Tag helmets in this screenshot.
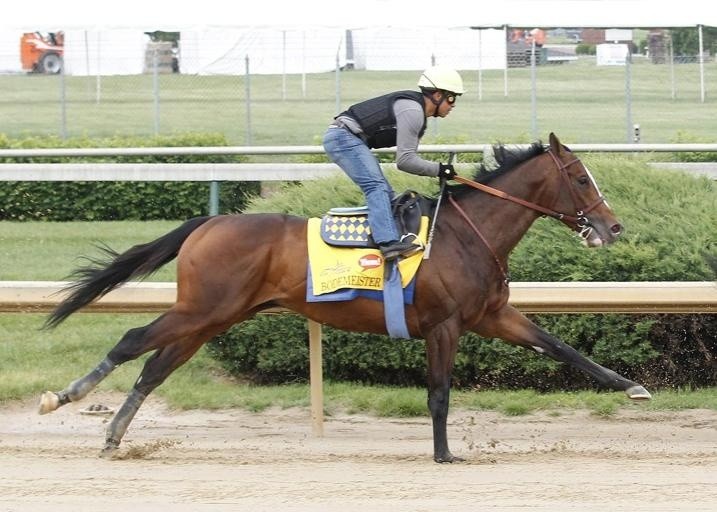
[418,65,467,97]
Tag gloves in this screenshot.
[441,165,458,180]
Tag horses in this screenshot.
[36,131,654,464]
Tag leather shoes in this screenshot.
[379,239,420,261]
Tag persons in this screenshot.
[322,67,465,264]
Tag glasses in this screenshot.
[445,92,456,103]
